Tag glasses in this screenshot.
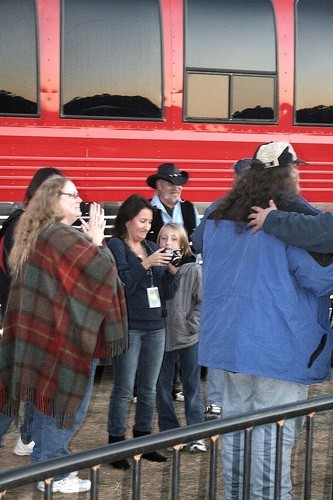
[59,192,80,199]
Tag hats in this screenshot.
[147,163,189,189]
[255,141,308,169]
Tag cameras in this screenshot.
[163,249,181,266]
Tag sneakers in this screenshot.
[13,435,36,455]
[36,471,92,494]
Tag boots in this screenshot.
[108,434,131,470]
[133,429,168,462]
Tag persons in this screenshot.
[196,140,333,500]
[0,156,252,470]
[246,200,333,255]
[0,177,127,492]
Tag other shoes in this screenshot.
[172,443,189,453]
[172,391,185,402]
[188,438,208,453]
